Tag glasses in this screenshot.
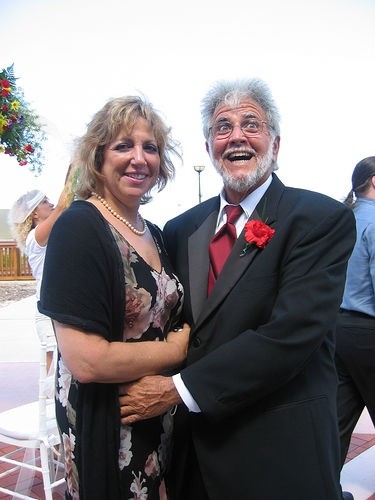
[209,118,274,139]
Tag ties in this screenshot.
[208,205,245,301]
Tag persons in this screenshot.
[118,76,357,500]
[37,95,191,500]
[7,154,79,374]
[334,158,375,500]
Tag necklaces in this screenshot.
[91,191,148,236]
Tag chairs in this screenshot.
[0,314,66,500]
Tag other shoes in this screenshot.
[341,491,354,500]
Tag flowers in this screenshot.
[239,196,276,258]
[0,61,50,178]
